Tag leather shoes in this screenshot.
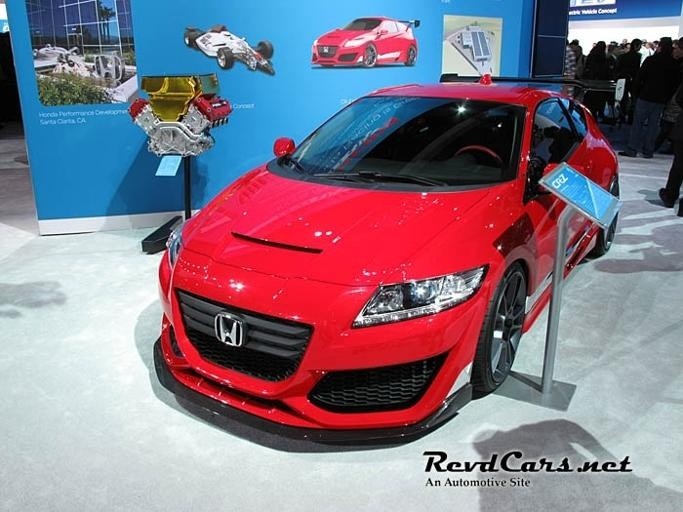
[659,188,674,207]
[618,152,653,158]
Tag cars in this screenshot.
[182,23,278,77]
[151,70,623,449]
[308,14,421,69]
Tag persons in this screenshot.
[564,37,683,157]
[58,54,74,65]
[660,90,683,207]
